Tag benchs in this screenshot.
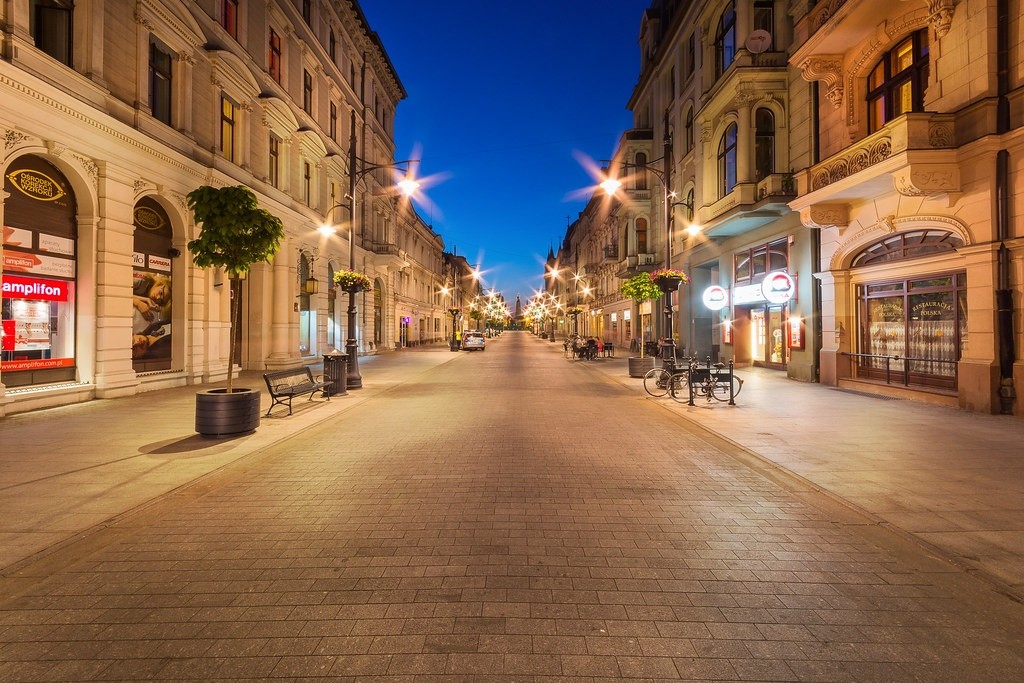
[262,366,334,416]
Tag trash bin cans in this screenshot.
[321,348,350,398]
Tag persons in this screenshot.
[563,334,604,360]
[456,330,462,350]
[132,272,172,360]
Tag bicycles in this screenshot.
[666,357,744,404]
[644,357,711,398]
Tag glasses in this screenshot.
[159,285,165,306]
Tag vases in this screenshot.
[653,276,682,292]
[341,285,361,293]
[449,309,459,314]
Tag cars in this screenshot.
[463,332,486,351]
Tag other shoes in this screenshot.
[587,358,589,360]
[591,358,592,359]
[578,355,583,358]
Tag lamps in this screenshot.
[295,254,318,298]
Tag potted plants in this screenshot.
[185,185,287,435]
[619,271,666,377]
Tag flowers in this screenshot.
[447,306,462,311]
[566,306,583,312]
[333,269,373,293]
[645,269,693,286]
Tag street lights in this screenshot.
[321,159,421,391]
[549,264,590,341]
[441,265,481,353]
[600,159,699,389]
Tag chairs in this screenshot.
[566,340,614,360]
